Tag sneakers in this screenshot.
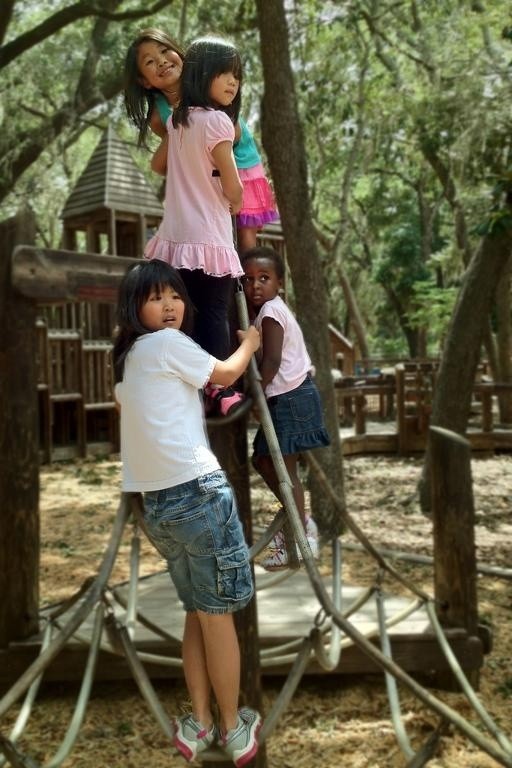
[201,381,246,417]
[263,512,321,571]
[172,707,263,768]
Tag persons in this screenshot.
[108,257,268,766]
[122,26,281,255]
[142,32,248,419]
[239,245,334,570]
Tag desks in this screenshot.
[335,372,512,432]
